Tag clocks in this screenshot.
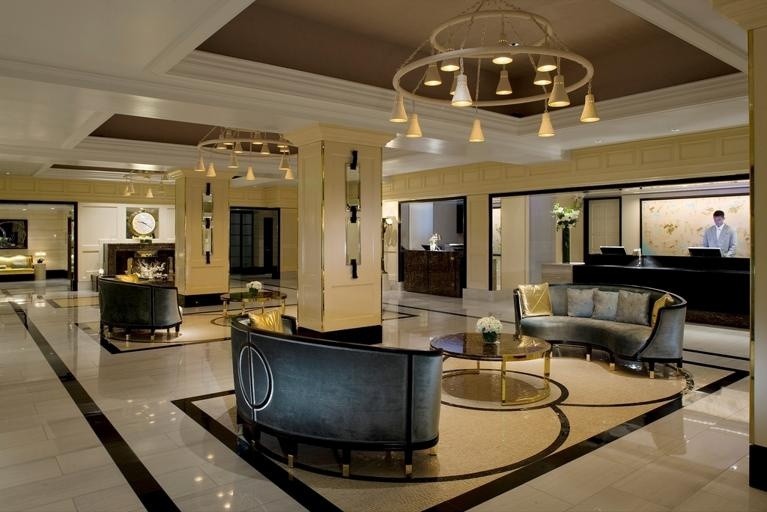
[126,207,156,238]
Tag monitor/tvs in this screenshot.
[600,246,627,255]
[688,247,722,257]
[422,244,430,249]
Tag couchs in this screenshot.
[0,253,33,278]
[98,275,182,341]
[514,281,690,381]
[228,309,443,477]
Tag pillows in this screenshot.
[591,289,618,319]
[115,274,137,285]
[613,288,651,325]
[246,308,283,333]
[517,282,554,319]
[650,292,672,327]
[566,286,599,317]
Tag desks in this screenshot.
[540,261,584,285]
[34,263,46,282]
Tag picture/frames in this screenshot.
[0,218,28,249]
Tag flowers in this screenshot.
[245,280,262,292]
[548,202,580,229]
[35,251,46,263]
[475,316,500,333]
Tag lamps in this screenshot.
[387,0,600,146]
[120,169,164,200]
[195,123,297,184]
[381,217,403,274]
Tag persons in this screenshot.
[703,211,737,257]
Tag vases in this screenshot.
[484,331,496,341]
[248,287,257,295]
[561,228,569,263]
